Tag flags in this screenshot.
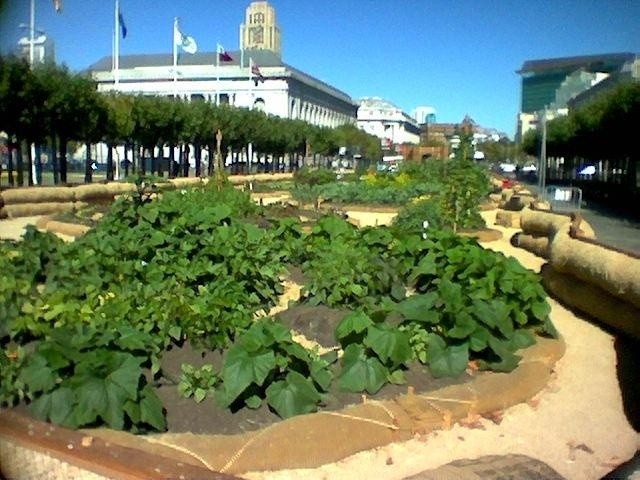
[120,12,127,38]
[175,25,197,55]
[218,45,233,62]
[53,0,61,13]
[252,62,264,86]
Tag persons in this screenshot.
[40,151,49,173]
[200,144,210,178]
[183,159,190,177]
[256,157,299,173]
[172,161,182,177]
